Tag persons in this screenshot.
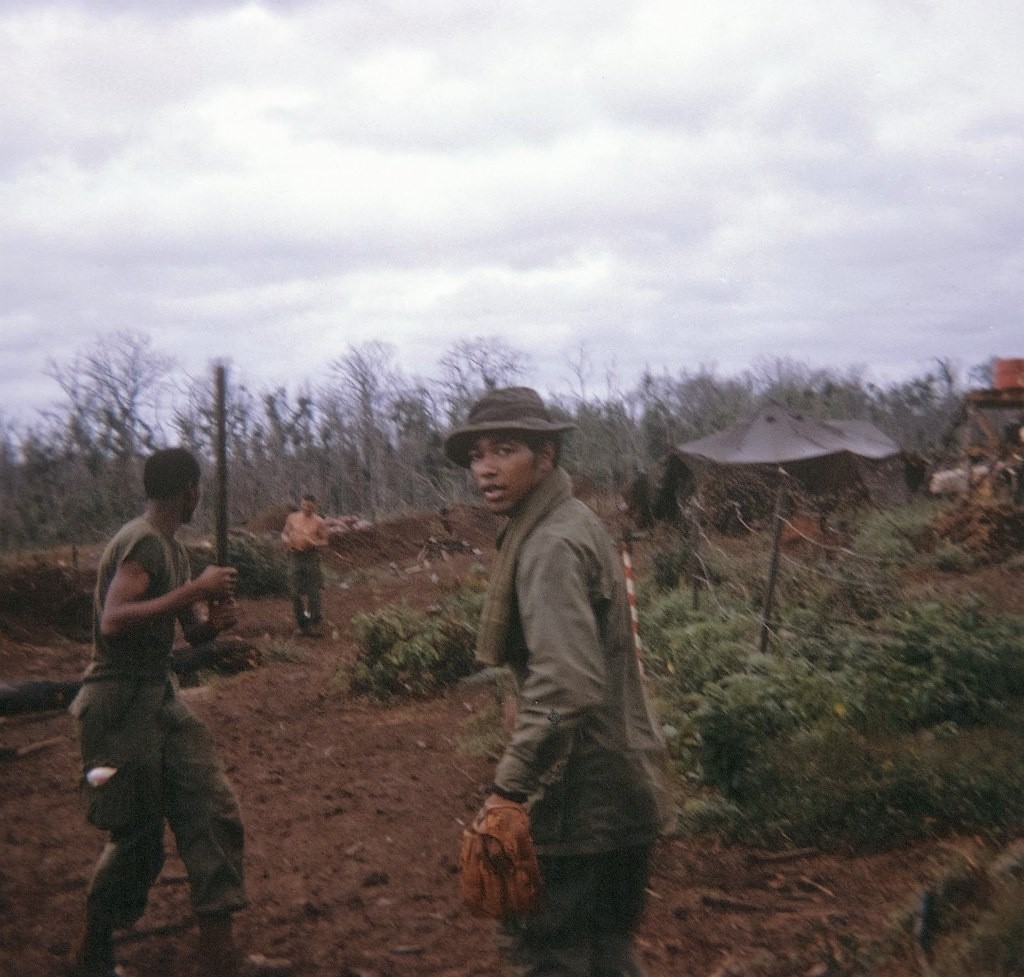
[280,495,330,639]
[445,389,674,976]
[73,448,291,976]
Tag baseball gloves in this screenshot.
[457,801,542,919]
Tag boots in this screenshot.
[73,892,141,977]
[196,913,292,977]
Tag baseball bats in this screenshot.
[214,362,228,568]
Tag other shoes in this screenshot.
[300,617,324,637]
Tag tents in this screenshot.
[676,395,902,467]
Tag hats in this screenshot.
[444,387,579,469]
